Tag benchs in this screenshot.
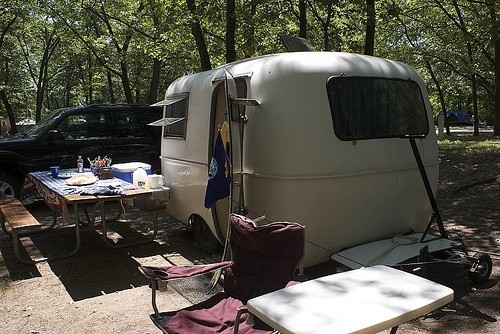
[119,198,168,245]
[0,198,41,264]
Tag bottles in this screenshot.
[98,167,113,179]
[77,156,83,173]
[132,167,148,190]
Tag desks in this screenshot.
[20,168,169,262]
[247,264,454,334]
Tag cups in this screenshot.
[50,166,60,178]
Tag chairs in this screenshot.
[138,213,305,334]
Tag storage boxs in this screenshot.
[110,162,152,184]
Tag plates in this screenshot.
[65,178,98,185]
[57,173,76,178]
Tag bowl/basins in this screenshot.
[149,174,165,188]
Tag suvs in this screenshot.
[0,103,163,201]
